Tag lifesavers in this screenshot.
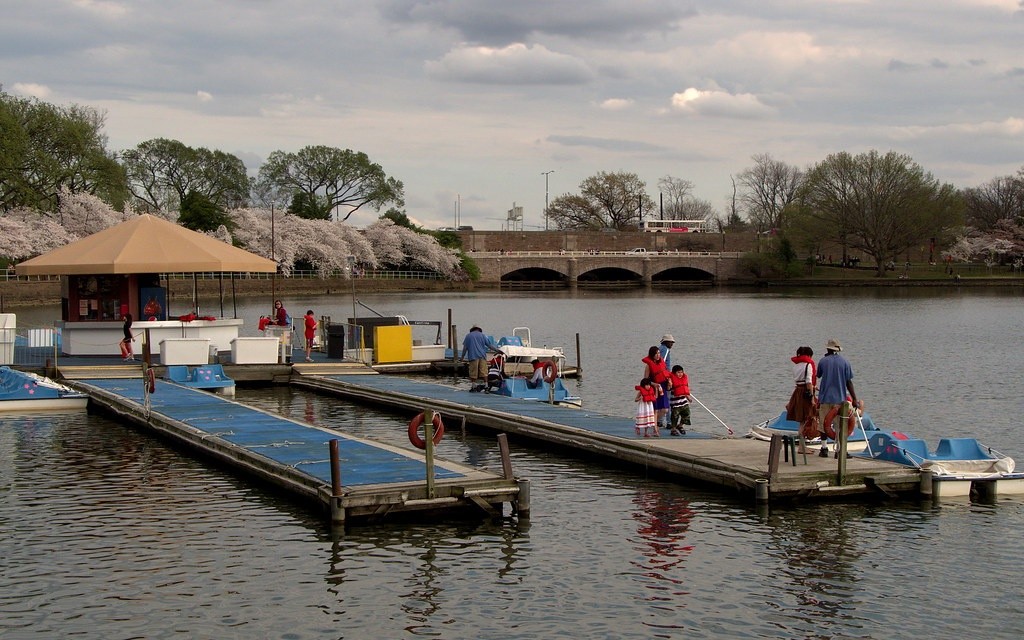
[542,360,557,383]
[148,368,155,393]
[823,405,855,441]
[408,411,444,449]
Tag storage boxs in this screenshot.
[229,337,281,364]
[158,338,211,365]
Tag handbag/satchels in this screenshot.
[802,405,821,440]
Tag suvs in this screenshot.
[456,225,473,232]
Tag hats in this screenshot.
[825,338,843,352]
[470,324,482,332]
[531,359,539,364]
[660,334,675,343]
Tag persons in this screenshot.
[304,310,320,363]
[815,253,861,269]
[528,360,550,385]
[634,335,693,438]
[658,247,742,255]
[121,313,136,361]
[559,248,601,255]
[885,255,1014,280]
[460,324,503,393]
[785,338,870,459]
[7,262,14,280]
[272,300,287,326]
[470,247,512,255]
[488,357,510,391]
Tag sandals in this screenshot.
[671,425,685,436]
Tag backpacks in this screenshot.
[280,308,291,327]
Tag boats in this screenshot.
[847,431,1024,496]
[488,345,581,408]
[748,394,881,453]
[0,365,89,410]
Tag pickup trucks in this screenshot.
[625,247,658,256]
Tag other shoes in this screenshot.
[469,388,477,392]
[834,451,852,459]
[798,446,815,454]
[818,447,828,457]
[653,430,660,437]
[304,357,314,363]
[657,421,672,429]
[123,353,134,361]
[485,388,489,394]
[643,433,650,438]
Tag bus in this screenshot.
[637,219,706,233]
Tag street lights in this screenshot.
[541,170,555,231]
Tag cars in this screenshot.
[763,229,787,235]
[438,227,456,232]
